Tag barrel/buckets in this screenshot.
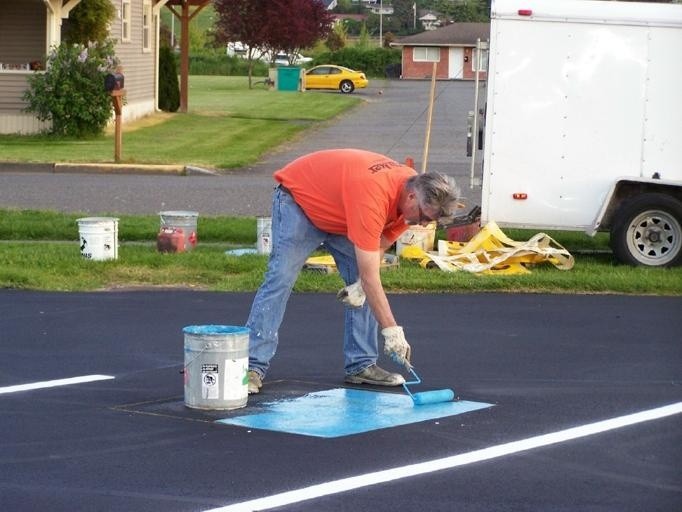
[179,324,251,413]
[74,215,118,264]
[447,220,483,243]
[253,214,274,255]
[157,210,198,253]
[394,220,438,259]
[276,65,301,92]
[268,67,277,91]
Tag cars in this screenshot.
[272,53,313,66]
[304,64,368,94]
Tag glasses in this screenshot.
[417,204,433,223]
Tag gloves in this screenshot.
[381,326,411,365]
[337,278,366,308]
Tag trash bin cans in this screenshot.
[277,67,300,92]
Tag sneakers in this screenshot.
[345,363,405,386]
[248,370,263,394]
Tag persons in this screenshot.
[243,147,460,394]
